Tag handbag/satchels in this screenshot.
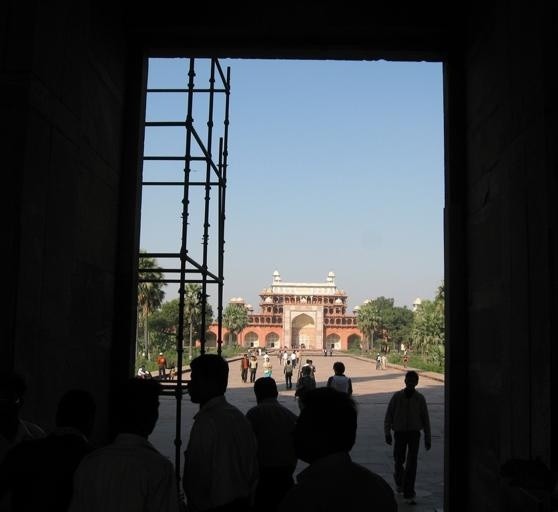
[295,383,305,396]
[264,370,269,374]
[287,372,292,376]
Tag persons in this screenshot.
[184,353,255,512]
[1,364,47,455]
[328,361,351,397]
[384,370,431,502]
[330,347,332,356]
[0,386,99,512]
[240,346,316,408]
[246,376,301,512]
[381,355,387,370]
[324,347,327,356]
[138,365,152,379]
[166,361,175,380]
[277,388,400,511]
[68,376,184,510]
[375,352,384,370]
[156,352,168,380]
